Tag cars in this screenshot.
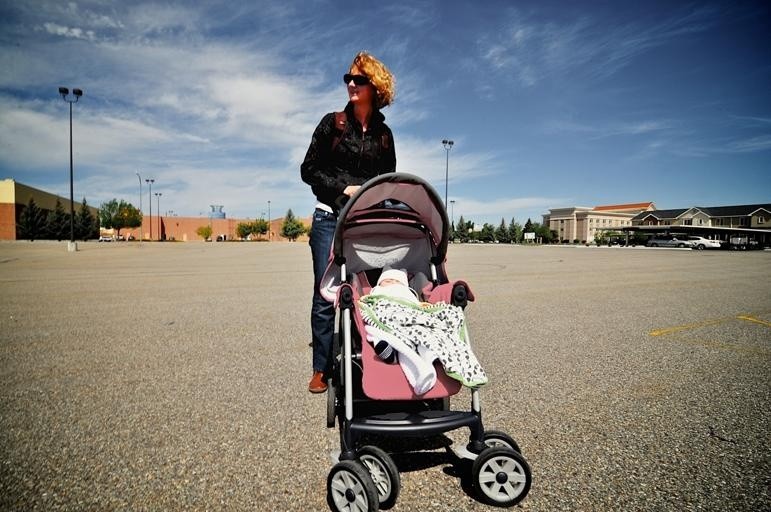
[100,234,135,242]
[448,238,484,243]
[646,233,758,250]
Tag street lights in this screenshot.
[442,140,454,211]
[169,210,179,240]
[268,200,271,241]
[59,86,84,253]
[247,212,266,240]
[146,179,155,242]
[450,200,456,242]
[136,173,142,244]
[156,193,162,241]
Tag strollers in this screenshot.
[319,172,532,511]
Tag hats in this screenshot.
[377,266,409,287]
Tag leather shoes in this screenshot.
[309,371,328,393]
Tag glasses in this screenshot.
[343,73,370,86]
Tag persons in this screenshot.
[364,263,419,365]
[301,51,397,394]
